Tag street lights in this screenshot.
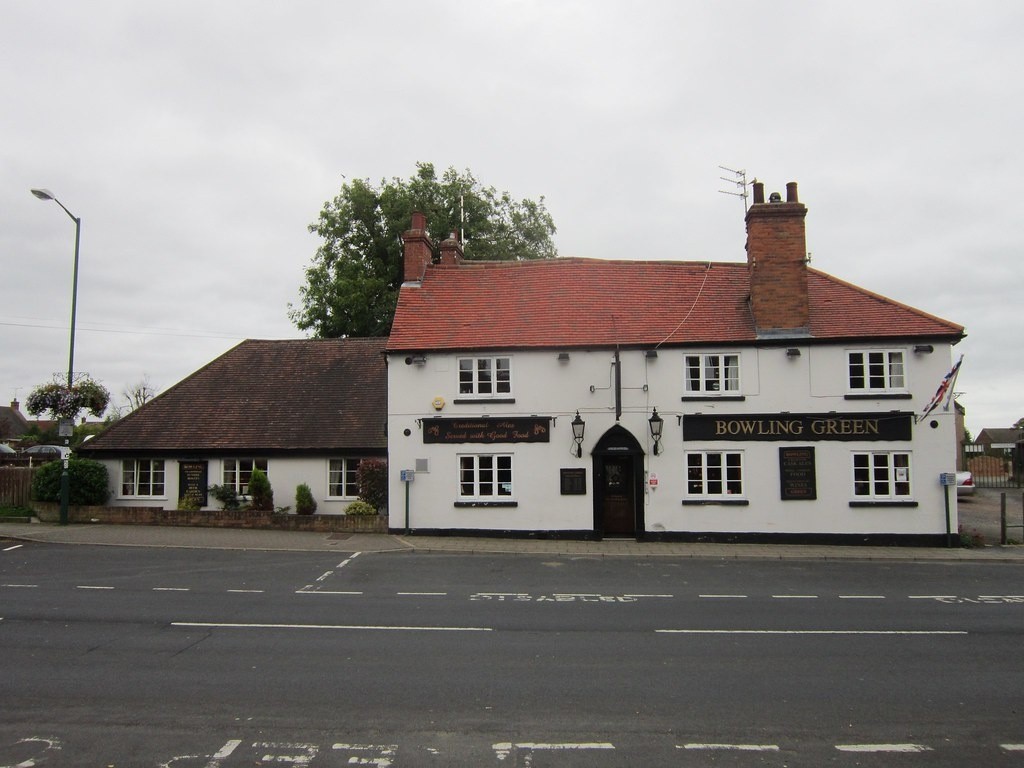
[31,186,81,524]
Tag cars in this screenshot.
[956,471,975,496]
[19,445,64,459]
[0,444,17,459]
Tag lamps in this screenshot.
[571,409,586,458]
[648,406,664,456]
[645,350,658,359]
[785,349,802,359]
[410,355,427,367]
[558,353,570,362]
[913,345,934,354]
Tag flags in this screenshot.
[920,363,960,421]
